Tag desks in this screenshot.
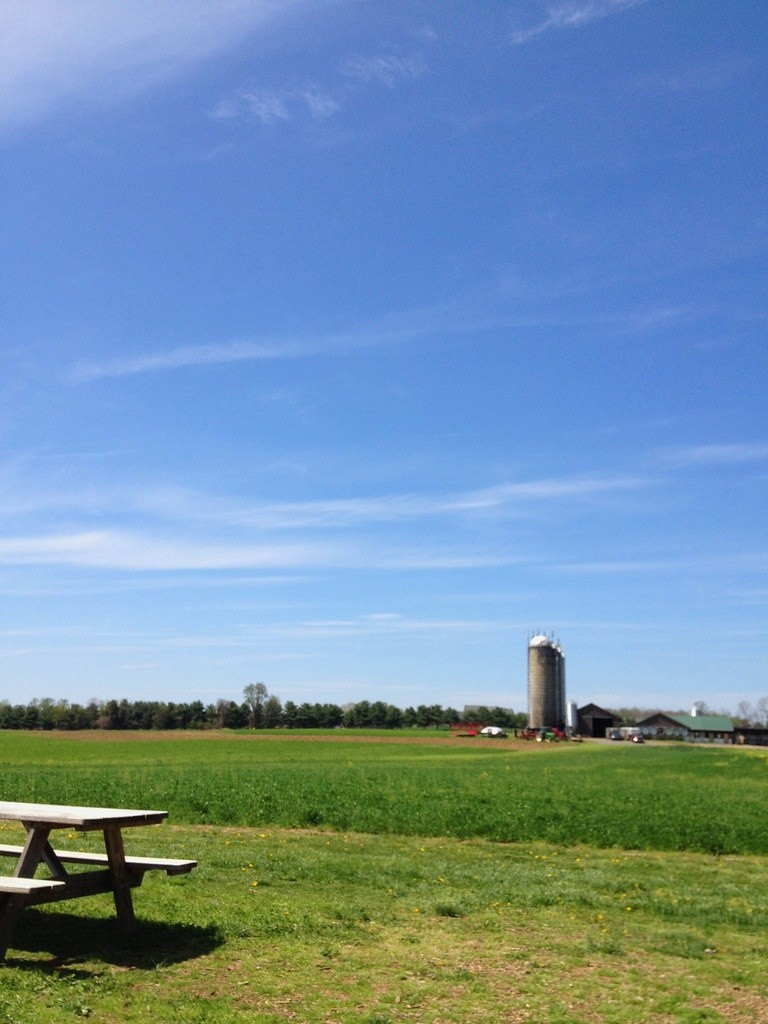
[0,800,198,964]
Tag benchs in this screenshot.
[0,844,198,876]
[0,876,66,894]
